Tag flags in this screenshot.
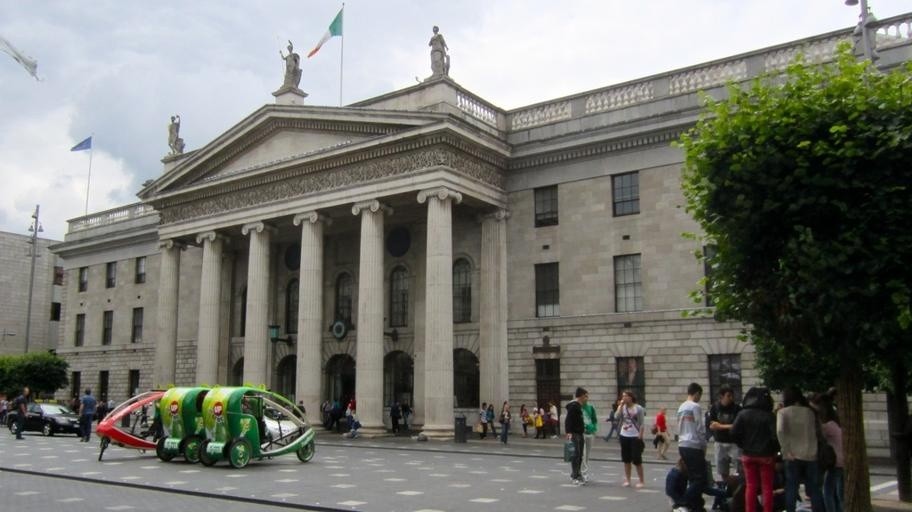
[308,6,344,60]
[70,137,92,152]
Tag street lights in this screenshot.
[22,198,47,354]
[0,332,19,337]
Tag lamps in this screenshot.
[268,322,293,346]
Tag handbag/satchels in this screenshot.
[499,410,504,424]
[476,423,484,433]
[819,445,836,464]
[652,427,658,434]
[564,441,577,464]
[524,416,532,424]
[398,418,405,425]
[536,416,543,428]
[346,409,351,416]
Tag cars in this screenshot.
[7,400,81,438]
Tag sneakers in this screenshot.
[601,436,609,442]
[570,476,646,488]
[15,436,24,439]
[522,432,559,440]
[657,454,667,460]
[80,436,90,442]
[480,435,501,440]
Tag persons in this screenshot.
[564,386,587,486]
[655,405,672,461]
[169,113,181,154]
[478,399,559,446]
[388,400,403,434]
[293,399,306,422]
[318,396,361,438]
[428,26,450,76]
[664,383,849,511]
[401,400,412,431]
[601,403,621,443]
[278,39,301,88]
[580,390,599,483]
[615,388,646,489]
[0,386,115,443]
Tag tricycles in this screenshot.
[90,383,320,471]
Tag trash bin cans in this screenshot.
[454,416,466,443]
